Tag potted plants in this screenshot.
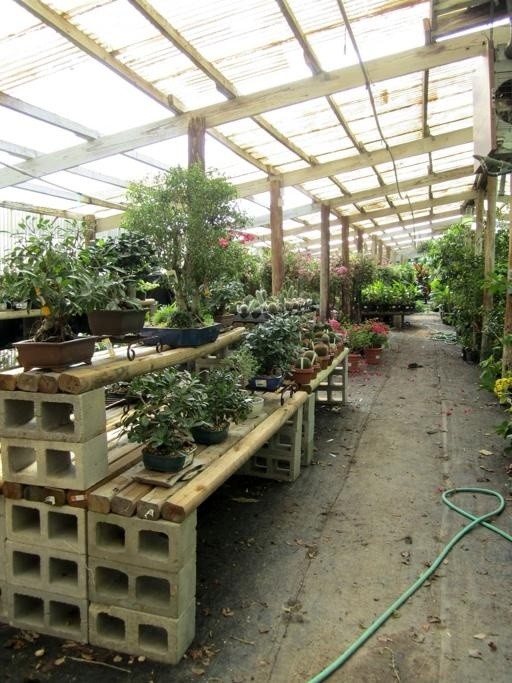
[429,226,490,366]
[0,153,416,473]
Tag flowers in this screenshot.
[494,377,512,440]
